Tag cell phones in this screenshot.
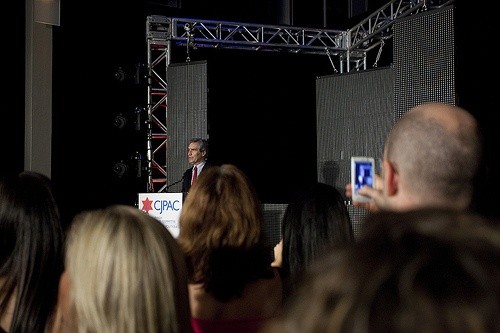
[350,156,376,203]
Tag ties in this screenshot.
[192,167,197,184]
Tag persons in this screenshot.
[289,102,500,333]
[0,164,500,333]
[181,138,211,202]
